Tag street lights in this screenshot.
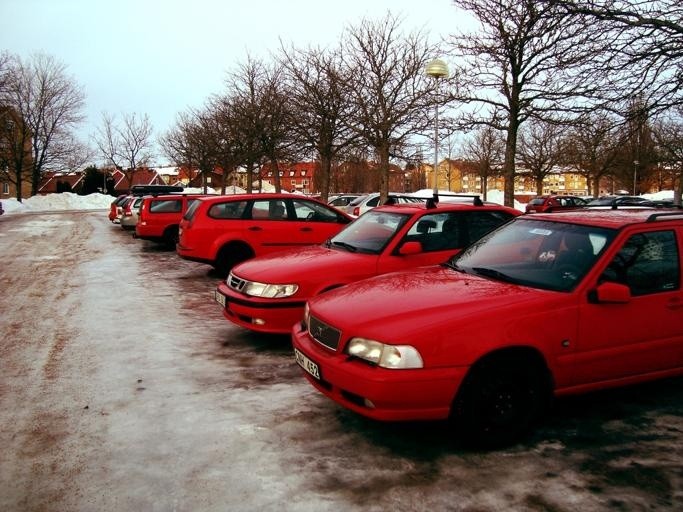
[423,60,448,200]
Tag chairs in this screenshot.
[545,231,589,271]
[269,205,286,219]
